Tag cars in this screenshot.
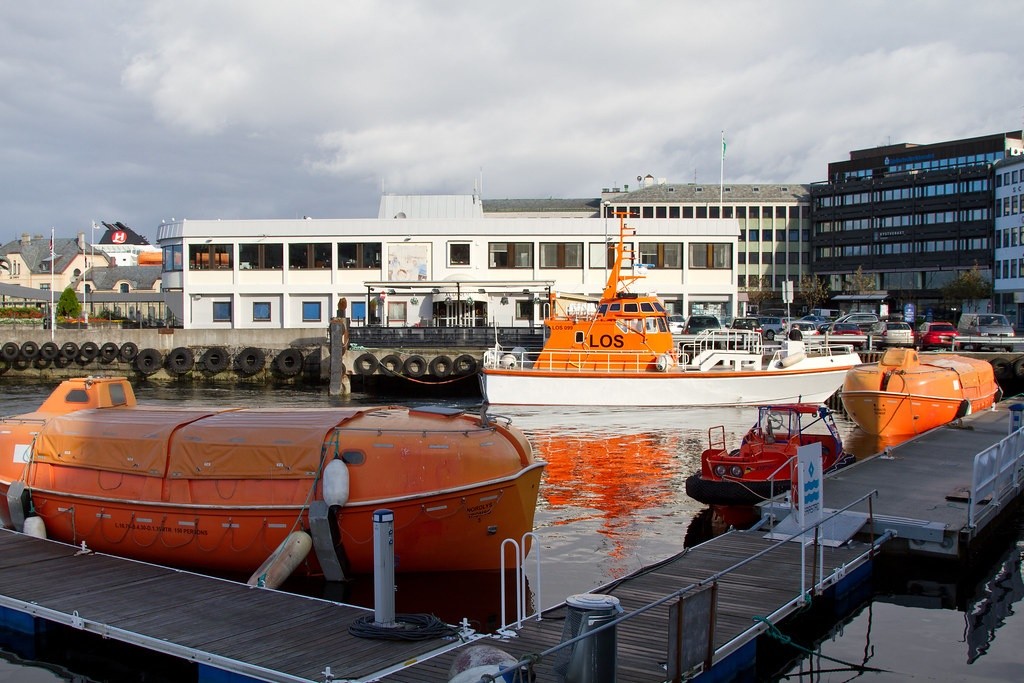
[824,323,864,351]
[726,317,765,348]
[779,322,822,352]
[914,322,960,352]
[742,313,830,341]
[867,320,914,350]
[667,314,685,334]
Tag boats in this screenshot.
[0,316,545,584]
[481,211,862,407]
[687,397,856,529]
[839,346,1002,438]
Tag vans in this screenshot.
[957,312,1016,352]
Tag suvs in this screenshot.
[818,312,882,336]
[677,314,722,350]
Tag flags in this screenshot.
[50,236,56,256]
[723,137,727,161]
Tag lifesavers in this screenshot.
[356,354,379,376]
[240,347,266,374]
[431,355,454,378]
[41,342,59,359]
[2,342,19,360]
[136,349,163,374]
[380,355,403,377]
[454,355,477,376]
[168,347,194,374]
[80,341,99,359]
[121,342,138,360]
[22,341,39,358]
[404,355,427,378]
[61,342,79,359]
[203,347,229,373]
[101,342,119,360]
[276,348,304,376]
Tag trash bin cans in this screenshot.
[552,593,625,683]
